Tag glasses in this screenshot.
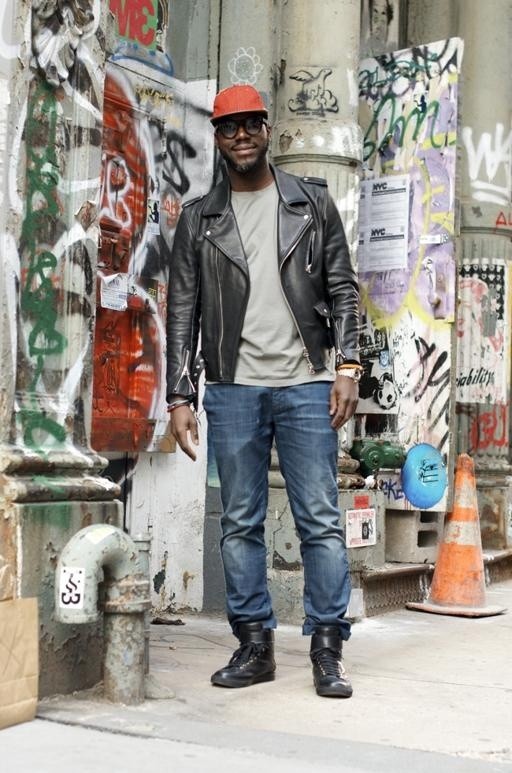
[213,112,271,140]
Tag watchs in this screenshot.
[336,369,362,383]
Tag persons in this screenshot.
[165,83,362,699]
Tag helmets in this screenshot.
[210,84,269,127]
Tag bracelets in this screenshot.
[337,363,365,377]
[167,399,189,413]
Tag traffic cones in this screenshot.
[404,452,507,617]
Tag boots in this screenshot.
[309,627,353,698]
[211,622,276,688]
